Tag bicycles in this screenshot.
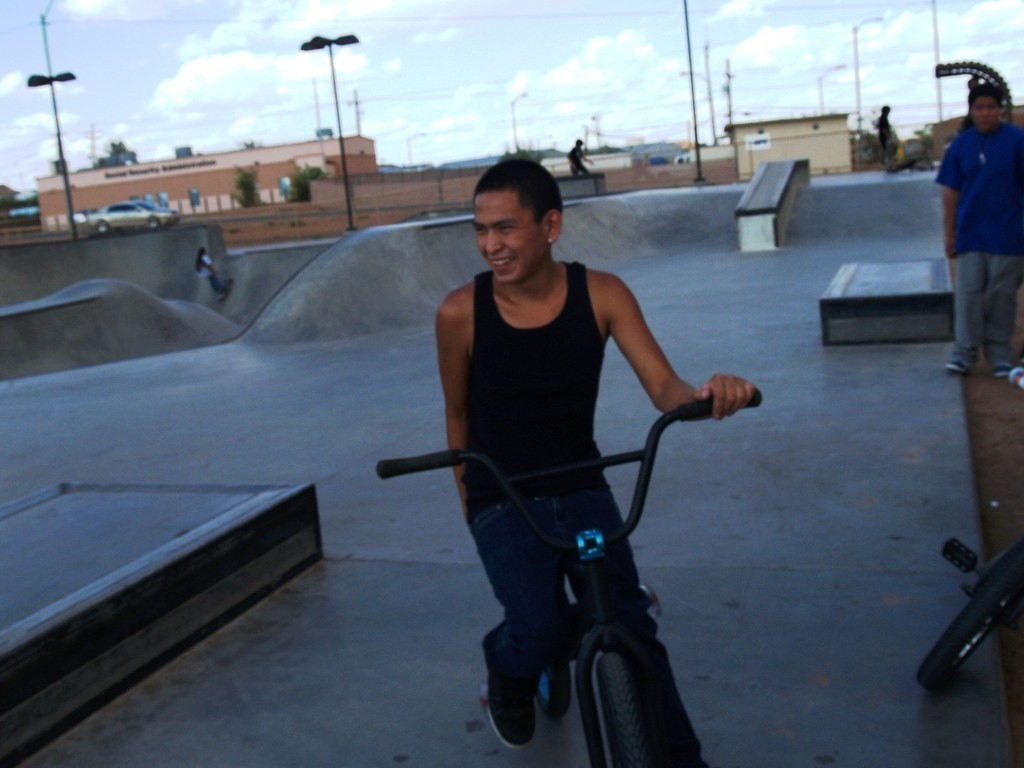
[375,389,764,764]
[911,530,1024,693]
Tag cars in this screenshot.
[86,198,181,234]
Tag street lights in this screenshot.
[302,35,361,230]
[28,72,78,241]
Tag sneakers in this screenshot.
[486,660,538,750]
[993,362,1012,378]
[945,360,967,374]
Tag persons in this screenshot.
[876,106,892,167]
[567,140,593,175]
[935,84,1024,376]
[195,247,231,301]
[435,159,753,768]
